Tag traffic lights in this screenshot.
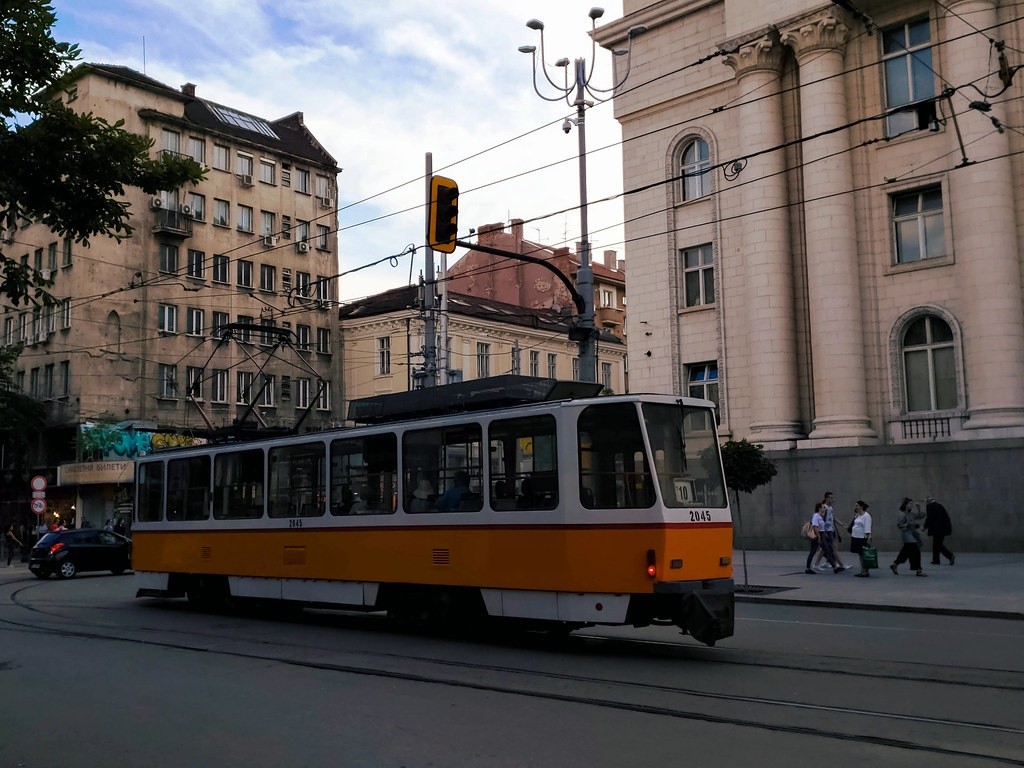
[429,175,460,253]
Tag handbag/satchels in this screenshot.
[800,521,817,539]
[848,515,858,534]
[913,532,923,547]
[862,539,879,569]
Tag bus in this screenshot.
[128,323,737,649]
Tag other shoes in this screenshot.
[805,568,817,574]
[890,564,899,575]
[916,569,927,577]
[8,564,14,568]
[854,574,870,577]
[834,566,844,573]
[950,556,955,565]
[931,561,940,564]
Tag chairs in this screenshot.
[287,477,540,517]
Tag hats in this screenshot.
[925,497,935,505]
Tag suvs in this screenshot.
[27,528,133,580]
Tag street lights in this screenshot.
[517,7,650,384]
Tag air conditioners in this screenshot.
[296,242,309,252]
[35,332,46,343]
[264,236,277,246]
[322,198,333,207]
[179,204,192,214]
[320,299,332,310]
[39,269,50,281]
[24,335,34,346]
[240,175,256,187]
[150,197,166,208]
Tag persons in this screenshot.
[805,491,955,577]
[5,517,126,568]
[229,470,547,519]
